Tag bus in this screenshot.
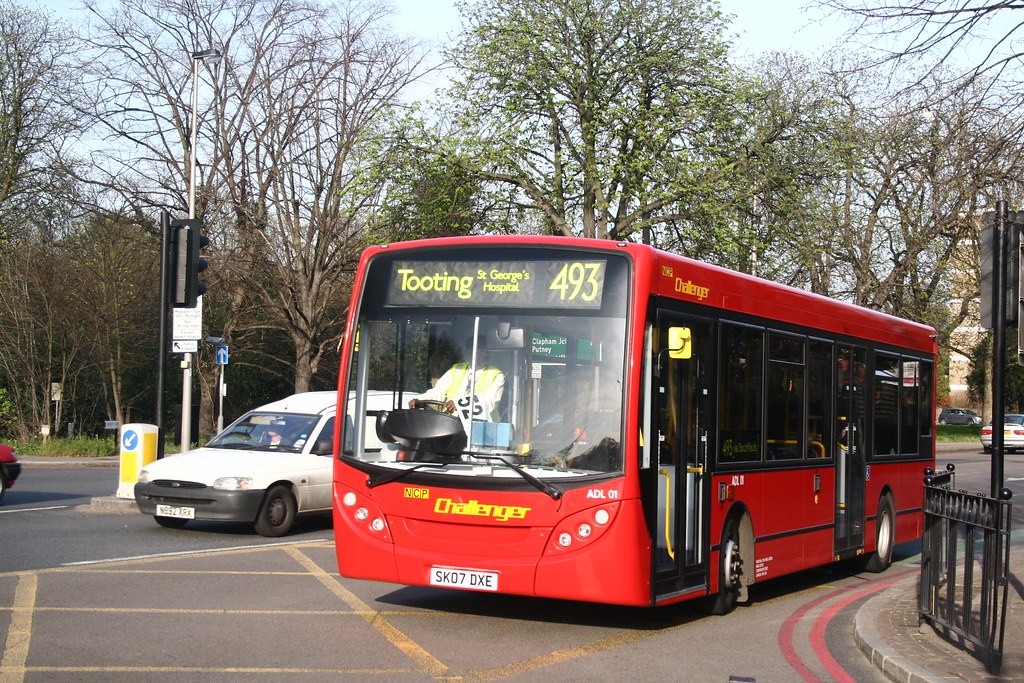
[330,233,939,617]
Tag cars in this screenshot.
[938,408,983,426]
[979,414,1024,455]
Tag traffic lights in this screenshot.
[172,219,209,309]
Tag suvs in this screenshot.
[133,389,421,536]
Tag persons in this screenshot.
[837,350,882,433]
[409,334,505,417]
[260,418,293,451]
